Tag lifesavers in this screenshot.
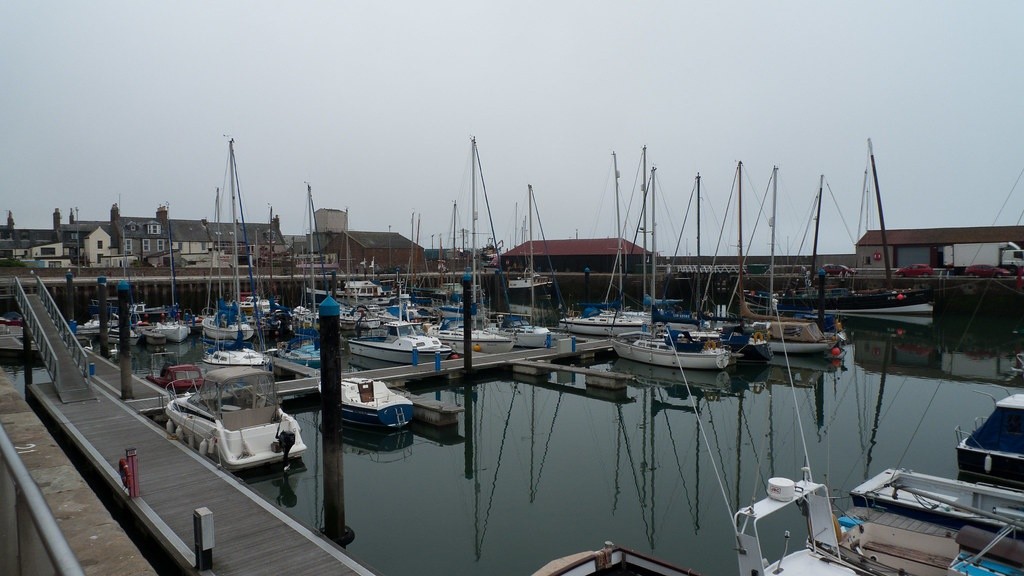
[754,332,763,341]
[302,320,313,330]
[119,459,130,488]
[706,341,716,350]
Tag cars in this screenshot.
[895,264,934,277]
[822,264,858,276]
[965,264,1011,279]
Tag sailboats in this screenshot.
[424,135,517,353]
[609,355,841,551]
[152,220,191,343]
[558,151,653,337]
[202,137,396,372]
[485,184,560,348]
[744,138,934,314]
[611,161,840,369]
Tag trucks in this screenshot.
[942,242,1024,275]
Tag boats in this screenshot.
[0,297,213,394]
[954,386,1024,490]
[348,321,452,364]
[531,541,703,576]
[313,410,414,463]
[165,365,414,472]
[850,468,1024,541]
[733,467,873,576]
